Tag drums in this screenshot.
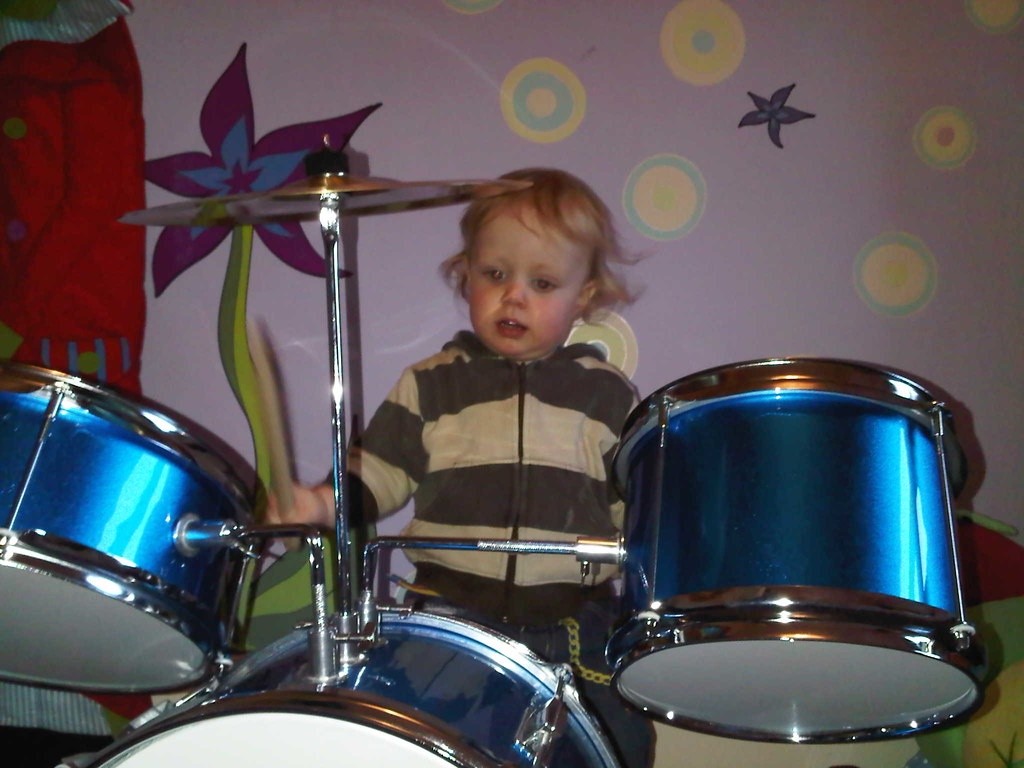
[604,355,991,746]
[64,601,618,768]
[1,359,268,694]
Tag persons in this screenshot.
[267,167,657,768]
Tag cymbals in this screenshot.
[116,171,537,228]
